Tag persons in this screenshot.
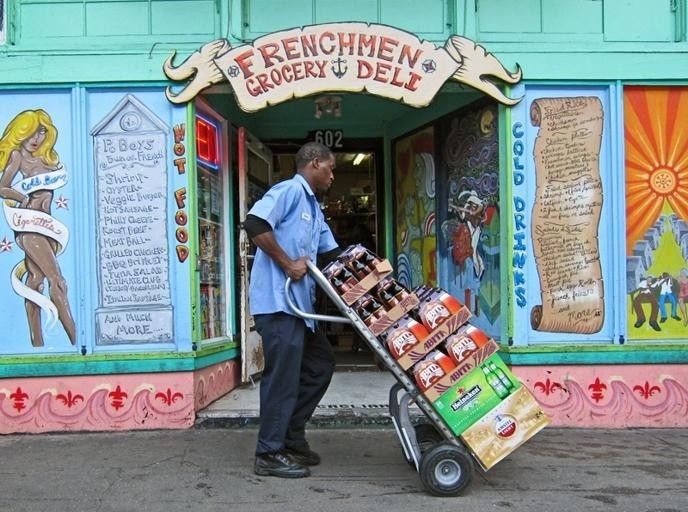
[244,142,343,478]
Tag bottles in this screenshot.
[323,242,410,327]
[479,360,514,400]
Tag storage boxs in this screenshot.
[459,382,550,470]
[432,354,522,436]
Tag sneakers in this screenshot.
[253,446,321,478]
